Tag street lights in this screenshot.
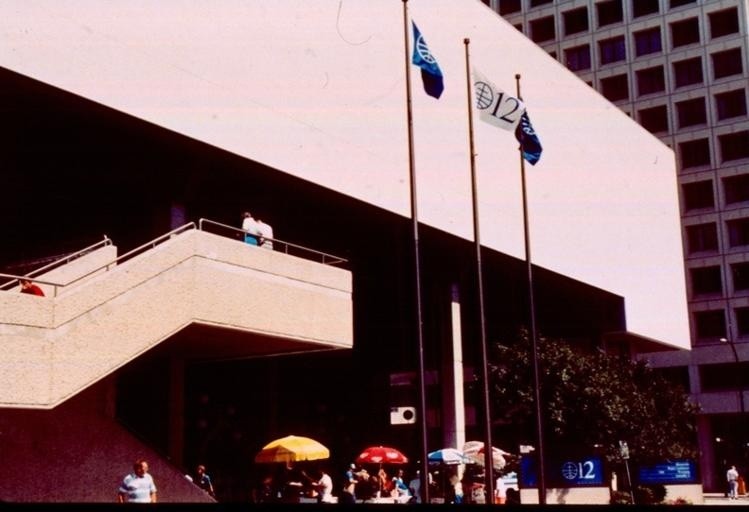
[720,337,745,457]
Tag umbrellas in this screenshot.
[354,444,409,498]
[253,434,332,470]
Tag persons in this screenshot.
[342,463,358,499]
[254,216,275,251]
[354,468,380,499]
[405,464,520,509]
[117,459,159,504]
[310,467,333,498]
[17,276,46,297]
[195,463,216,499]
[375,469,394,496]
[725,464,741,500]
[391,468,413,498]
[279,461,305,497]
[235,210,264,246]
[249,471,275,504]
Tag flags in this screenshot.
[515,103,544,170]
[467,61,522,132]
[410,16,445,101]
